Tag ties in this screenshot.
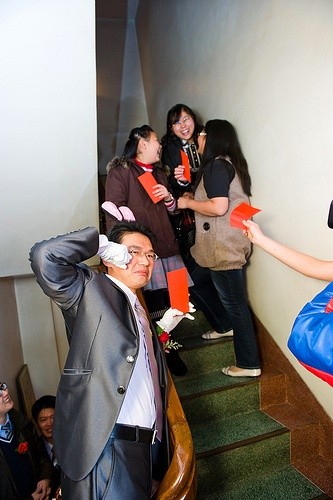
[1,421,12,439]
[135,296,163,443]
[51,446,60,470]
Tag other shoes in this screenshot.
[201,329,234,340]
[221,365,262,378]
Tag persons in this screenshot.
[104,103,263,377]
[0,381,62,500]
[28,201,196,500]
[243,200,333,388]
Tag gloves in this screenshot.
[99,234,132,270]
[156,293,197,334]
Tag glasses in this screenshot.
[0,382,8,397]
[172,116,193,127]
[129,248,160,262]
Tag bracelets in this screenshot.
[164,194,173,202]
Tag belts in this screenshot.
[110,423,158,446]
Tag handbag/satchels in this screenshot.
[174,208,195,249]
